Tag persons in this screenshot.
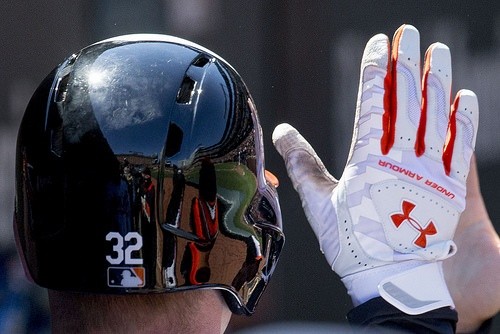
[113,150,219,286]
[443,153,499,334]
[13,25,481,334]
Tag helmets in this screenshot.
[9,31,284,319]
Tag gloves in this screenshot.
[265,22,481,318]
